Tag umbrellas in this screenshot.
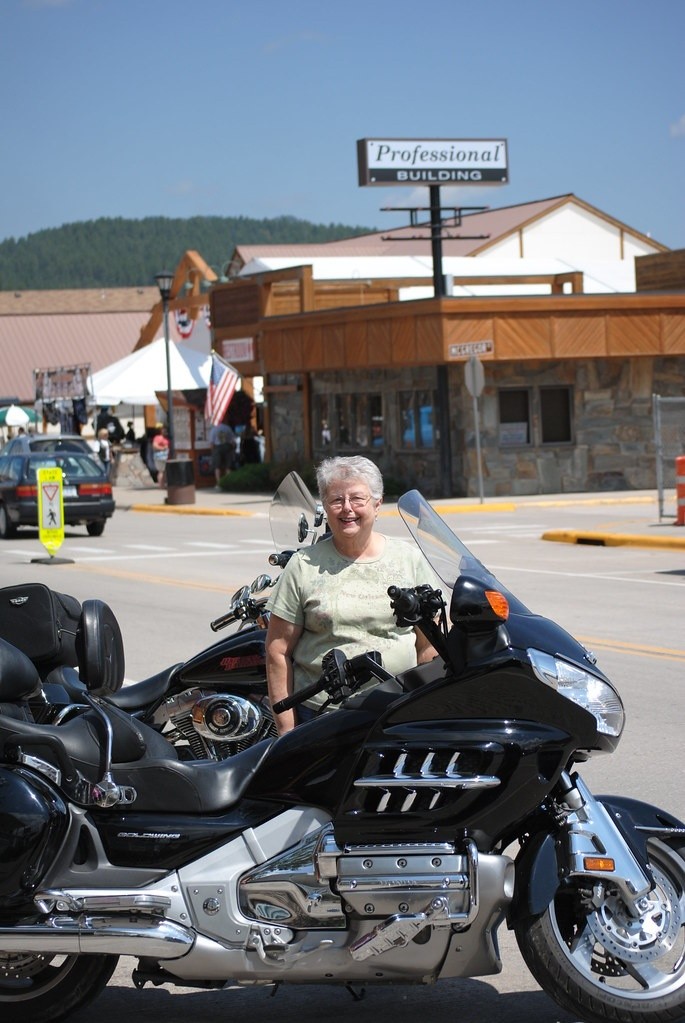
[0,404,44,427]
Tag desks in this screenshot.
[111,447,142,487]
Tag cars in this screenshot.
[345,404,439,450]
[0,433,109,479]
[0,454,116,537]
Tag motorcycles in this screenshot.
[0,470,685,1023]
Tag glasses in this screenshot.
[325,495,373,515]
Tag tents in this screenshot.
[84,337,264,406]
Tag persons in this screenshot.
[126,422,135,446]
[211,418,236,488]
[240,420,265,464]
[265,454,444,737]
[92,405,125,462]
[96,427,114,480]
[152,420,170,488]
[17,427,27,438]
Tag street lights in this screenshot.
[155,262,176,461]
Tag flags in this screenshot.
[204,356,241,428]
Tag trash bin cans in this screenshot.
[163,458,197,504]
